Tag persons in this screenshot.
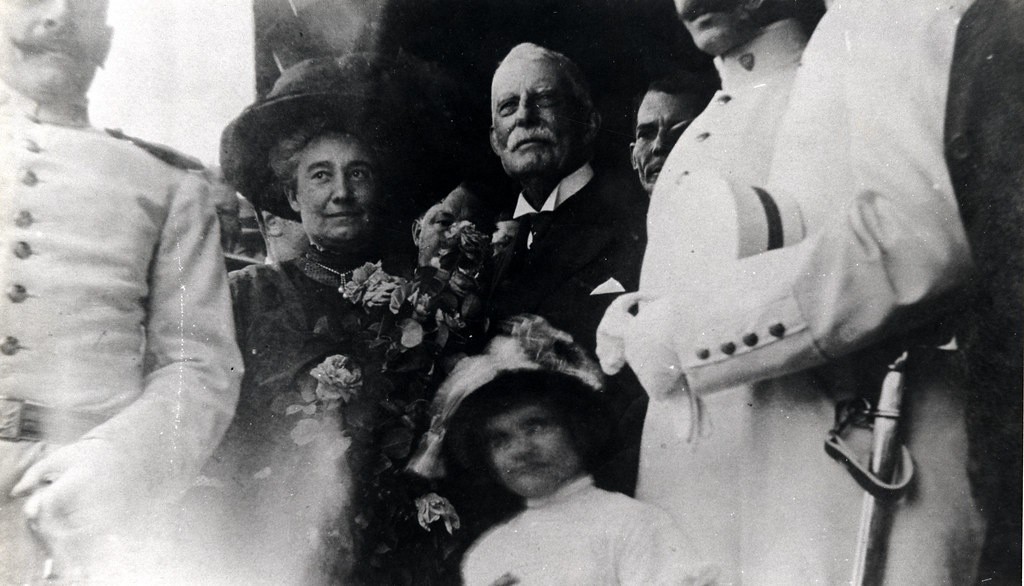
[200,180,254,273]
[0,1,246,586]
[410,316,721,586]
[628,1,811,586]
[218,55,446,586]
[254,205,311,271]
[597,1,991,586]
[399,39,645,586]
[603,64,719,505]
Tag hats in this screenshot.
[405,312,603,478]
[630,172,807,315]
[218,53,420,222]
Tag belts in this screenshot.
[0,391,116,444]
